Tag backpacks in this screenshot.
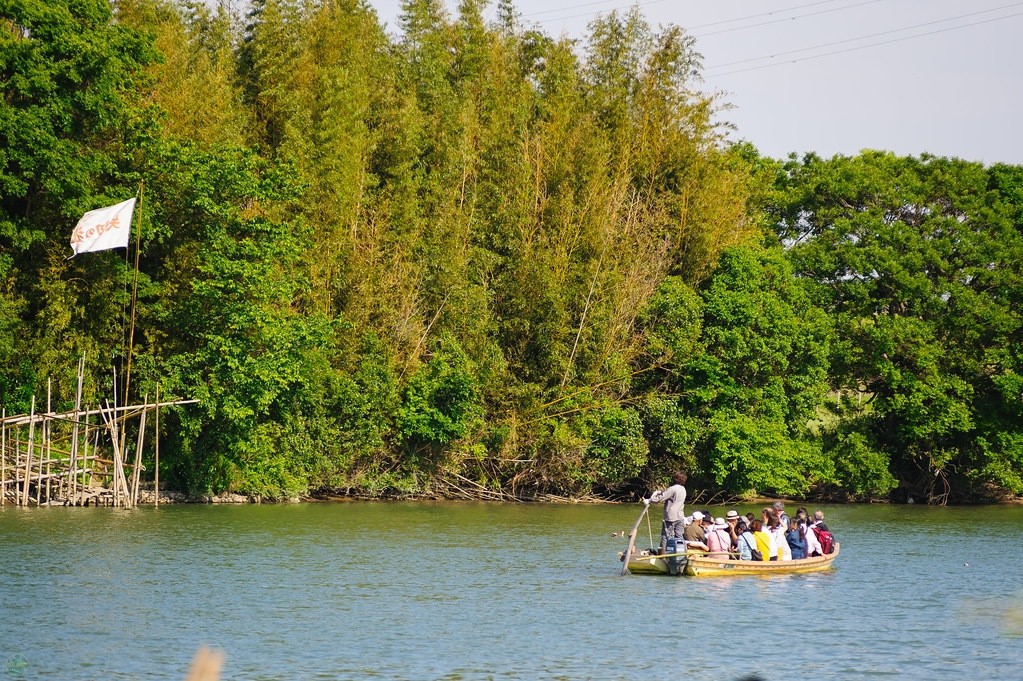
[812,528,834,554]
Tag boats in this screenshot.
[623,541,840,576]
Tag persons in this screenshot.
[683,502,835,562]
[652,472,686,560]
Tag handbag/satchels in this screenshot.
[751,549,763,560]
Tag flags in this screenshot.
[67,198,134,260]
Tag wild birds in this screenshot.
[611,531,625,537]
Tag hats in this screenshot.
[692,511,706,520]
[725,510,739,520]
[703,514,714,523]
[714,518,729,528]
